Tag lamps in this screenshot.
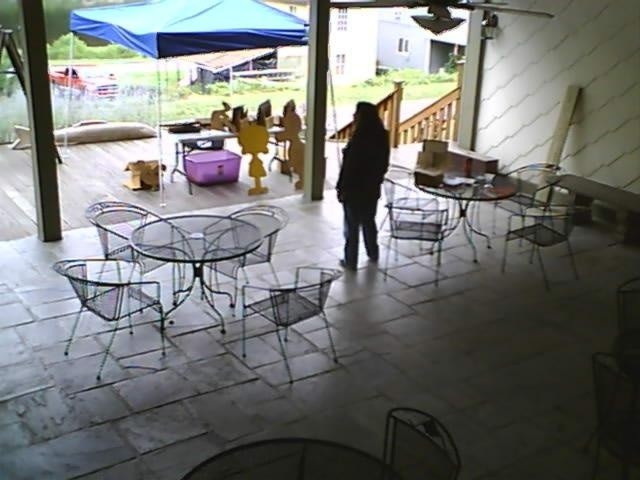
[479,12,504,42]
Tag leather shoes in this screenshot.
[339,258,357,272]
[371,252,380,262]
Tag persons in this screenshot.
[336,101,390,272]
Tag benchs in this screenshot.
[551,173,639,246]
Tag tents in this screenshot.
[64,0,341,207]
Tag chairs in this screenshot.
[382,196,450,289]
[241,266,346,385]
[51,257,167,381]
[614,276,640,336]
[83,199,193,316]
[376,164,422,246]
[200,204,291,318]
[382,407,462,480]
[501,203,581,293]
[491,168,562,266]
[590,351,640,479]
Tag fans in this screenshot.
[328,0,556,37]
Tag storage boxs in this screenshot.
[183,148,242,188]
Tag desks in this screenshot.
[169,124,293,195]
[180,437,406,480]
[129,214,265,336]
[415,170,521,265]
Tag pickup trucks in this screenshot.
[48,63,119,101]
